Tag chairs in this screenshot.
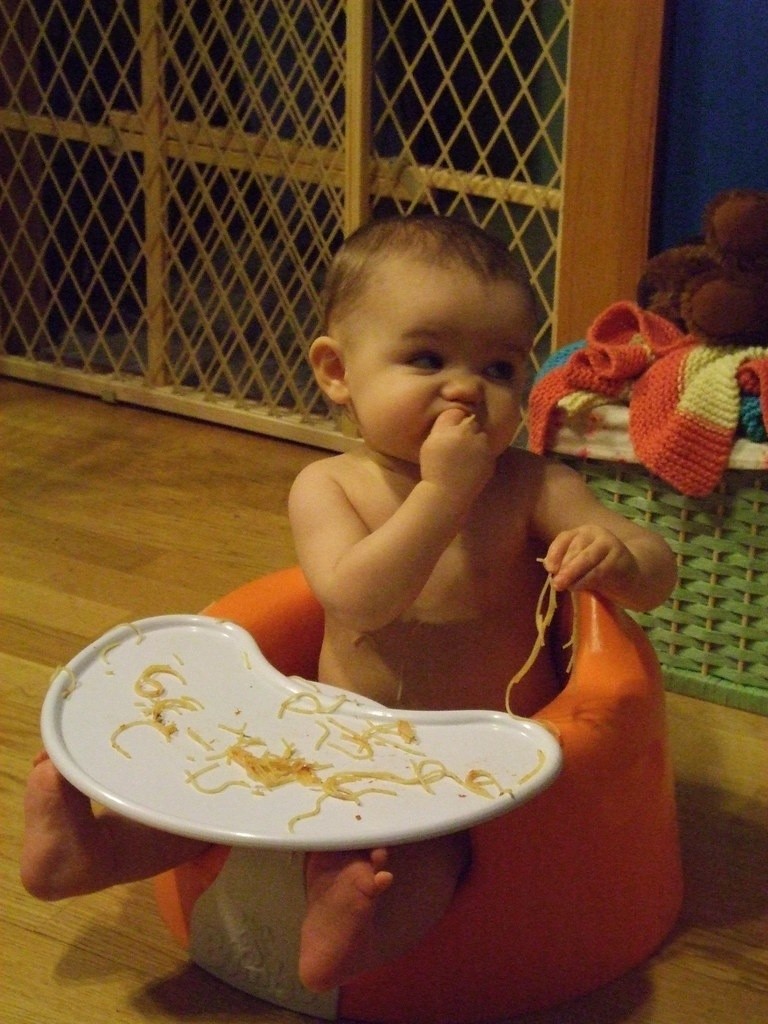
[37,562,684,1024]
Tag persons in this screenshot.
[19,212,678,994]
